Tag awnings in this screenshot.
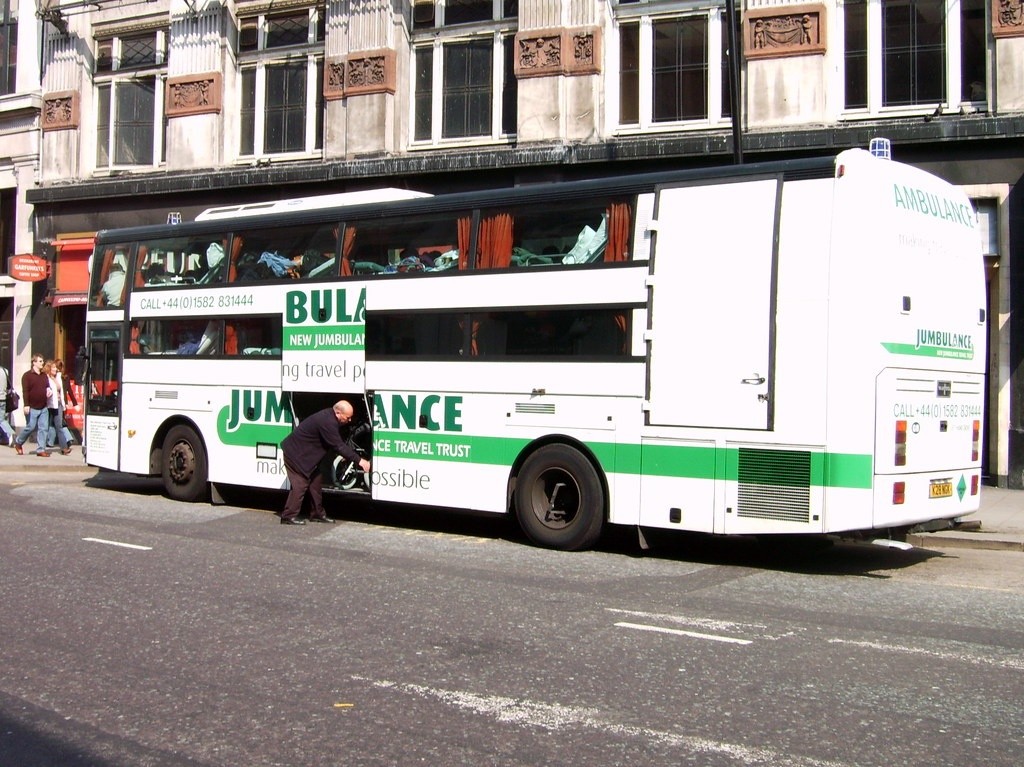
[52,291,87,308]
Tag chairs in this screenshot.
[355,249,459,275]
[149,242,224,286]
[299,257,337,280]
[512,216,606,266]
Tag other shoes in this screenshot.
[67,441,72,447]
[37,452,49,456]
[46,444,51,447]
[15,444,23,455]
[9,433,17,447]
[62,448,71,454]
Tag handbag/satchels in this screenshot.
[6,392,20,412]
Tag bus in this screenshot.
[73,148,986,553]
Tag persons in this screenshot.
[0,366,17,448]
[13,353,51,457]
[97,264,126,307]
[280,400,370,525]
[38,358,78,454]
[177,245,577,360]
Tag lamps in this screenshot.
[257,157,271,167]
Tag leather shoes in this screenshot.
[281,516,306,525]
[310,515,335,523]
